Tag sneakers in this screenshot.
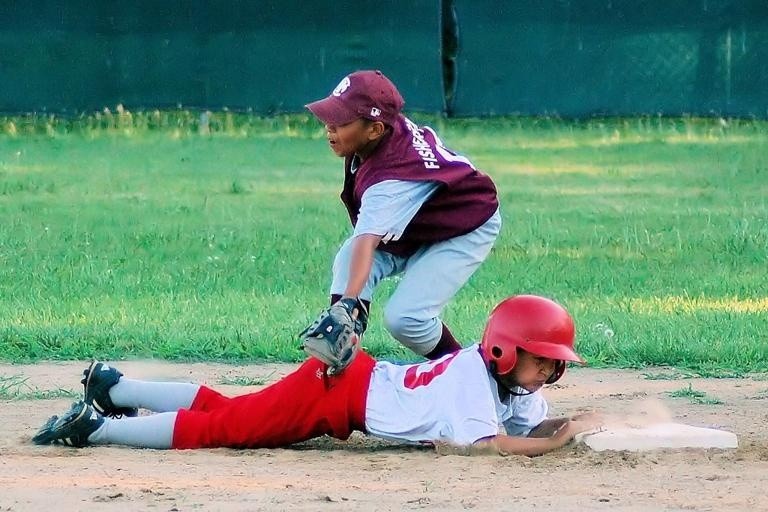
[80,359,139,420]
[31,399,106,449]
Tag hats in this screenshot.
[479,294,587,377]
[303,69,406,129]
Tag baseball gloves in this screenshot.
[298,296,368,374]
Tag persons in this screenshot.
[33,291,606,457]
[299,68,504,380]
